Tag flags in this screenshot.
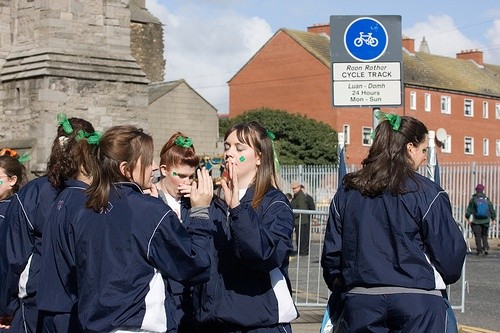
[338,148,347,191]
[320,109,469,333]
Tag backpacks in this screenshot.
[474,194,489,218]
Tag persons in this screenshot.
[0,113,315,333]
[465,185,497,255]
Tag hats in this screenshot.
[475,183,485,192]
[291,182,301,188]
